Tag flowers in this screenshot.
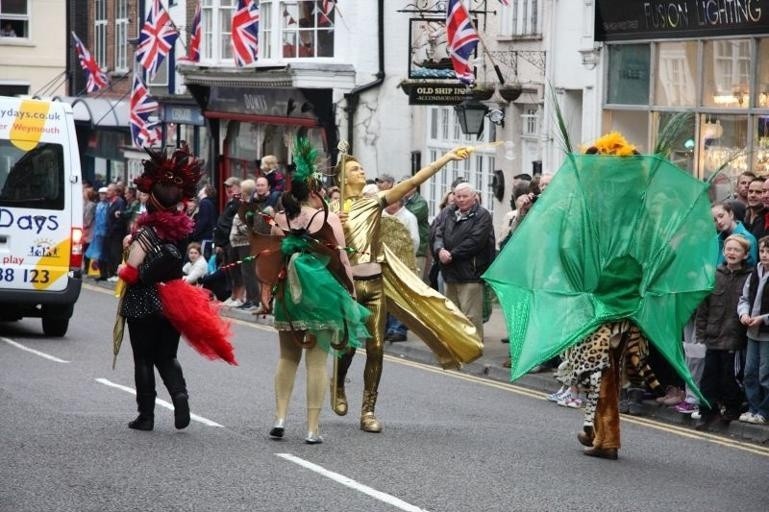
[573,128,637,160]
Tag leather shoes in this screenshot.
[577,429,619,459]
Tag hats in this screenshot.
[97,187,109,193]
[224,176,243,188]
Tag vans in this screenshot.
[0,95,86,339]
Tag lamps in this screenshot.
[451,80,507,135]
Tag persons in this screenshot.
[83,145,768,460]
[0,23,17,36]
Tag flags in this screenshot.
[129,75,162,150]
[446,0,479,86]
[177,3,202,63]
[76,40,112,93]
[231,0,260,67]
[136,0,180,81]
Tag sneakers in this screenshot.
[218,296,270,315]
[306,432,326,445]
[546,383,587,408]
[173,391,190,430]
[269,420,284,437]
[128,411,154,430]
[657,385,767,425]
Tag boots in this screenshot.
[330,377,348,415]
[628,388,647,413]
[358,390,382,433]
